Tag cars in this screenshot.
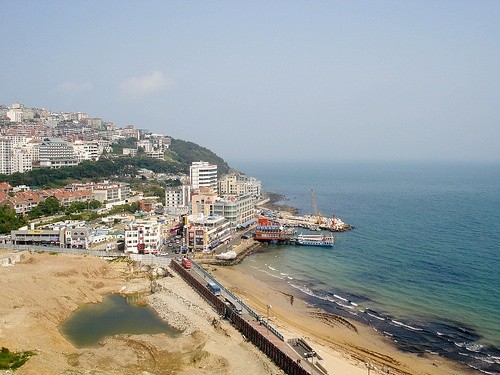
[168,243,172,247]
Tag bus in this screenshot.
[206,282,221,296]
[175,239,180,244]
[182,258,191,268]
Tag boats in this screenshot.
[294,232,335,247]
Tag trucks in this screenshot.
[224,297,242,315]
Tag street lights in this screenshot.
[265,304,272,336]
